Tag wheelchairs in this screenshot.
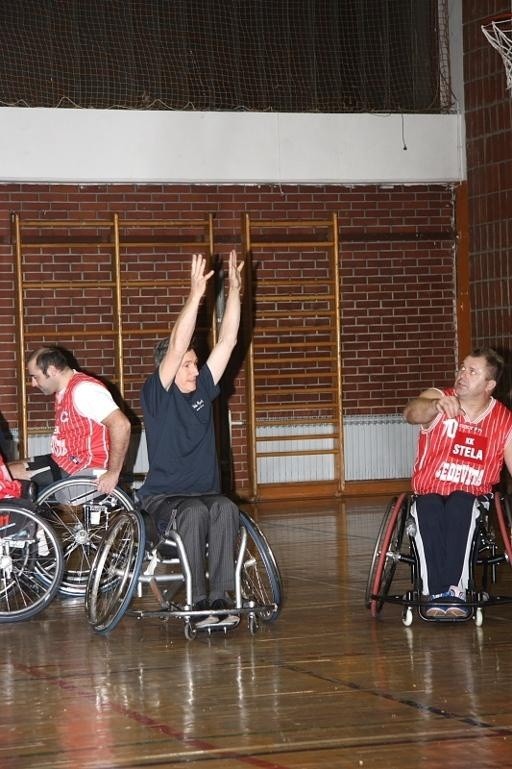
[365,491,512,626]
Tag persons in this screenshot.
[142,247,245,632]
[399,345,511,620]
[0,344,134,599]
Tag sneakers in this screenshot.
[212,598,241,631]
[189,599,220,626]
[424,591,445,617]
[446,591,468,617]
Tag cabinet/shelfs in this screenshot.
[14,213,349,503]
